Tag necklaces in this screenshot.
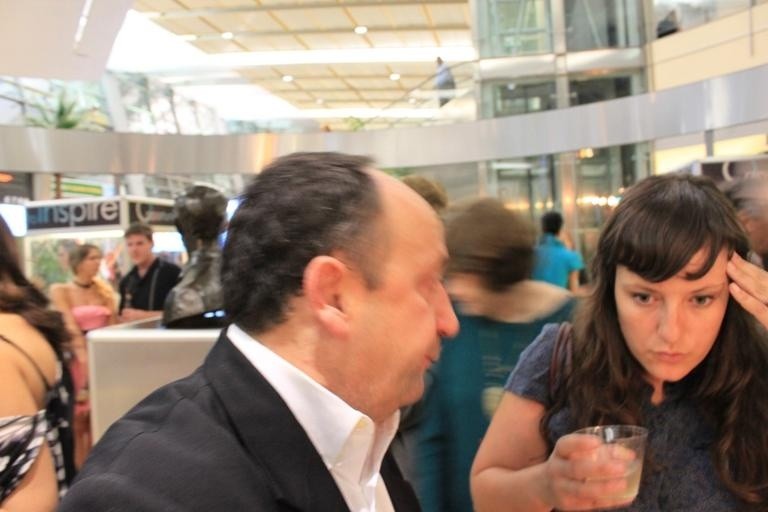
[72,277,94,289]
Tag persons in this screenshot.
[655,9,683,41]
[717,175,768,271]
[57,151,461,510]
[50,243,119,473]
[389,196,585,512]
[435,57,456,109]
[114,226,185,325]
[1,213,78,512]
[469,173,767,511]
[529,209,584,295]
[399,175,449,220]
[161,184,228,325]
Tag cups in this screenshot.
[581,422,647,500]
